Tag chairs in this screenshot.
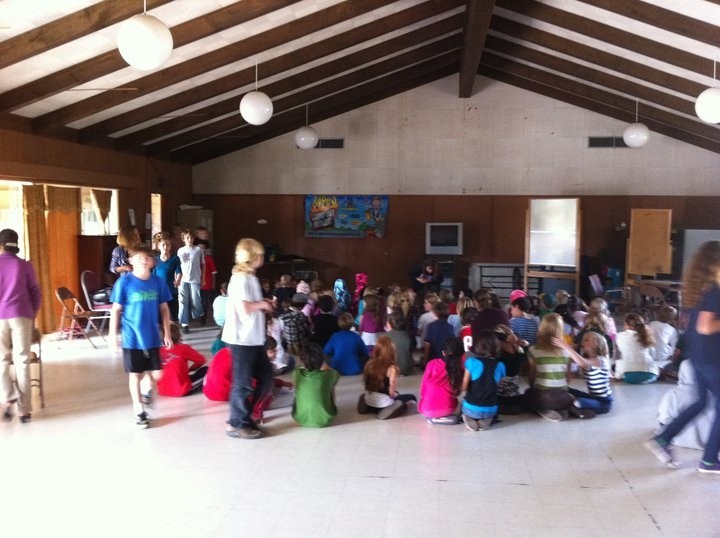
[80,270,125,337]
[639,285,678,329]
[589,274,627,316]
[7,329,45,411]
[54,287,108,350]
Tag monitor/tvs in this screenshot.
[425,222,464,255]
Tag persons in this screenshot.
[655,354,716,455]
[201,343,238,402]
[210,327,231,351]
[151,238,184,345]
[149,231,170,260]
[211,282,235,323]
[261,243,698,443]
[154,322,209,401]
[643,238,720,476]
[169,223,186,256]
[196,240,218,327]
[107,241,178,430]
[171,228,207,335]
[218,237,277,441]
[0,226,43,425]
[194,224,214,255]
[109,224,149,277]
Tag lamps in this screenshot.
[694,45,720,125]
[116,0,173,71]
[239,56,273,126]
[622,97,650,149]
[295,103,319,151]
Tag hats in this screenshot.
[296,280,311,294]
[0,229,20,253]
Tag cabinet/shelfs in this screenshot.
[178,209,214,253]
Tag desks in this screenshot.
[260,257,308,279]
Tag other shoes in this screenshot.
[378,400,407,419]
[228,423,264,439]
[200,311,206,326]
[182,326,189,334]
[20,415,31,423]
[4,408,12,421]
[536,410,562,422]
[141,391,152,404]
[135,411,150,429]
[358,393,369,414]
[462,414,478,432]
[476,418,493,429]
[427,415,458,425]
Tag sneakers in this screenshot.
[698,458,720,474]
[646,438,676,468]
